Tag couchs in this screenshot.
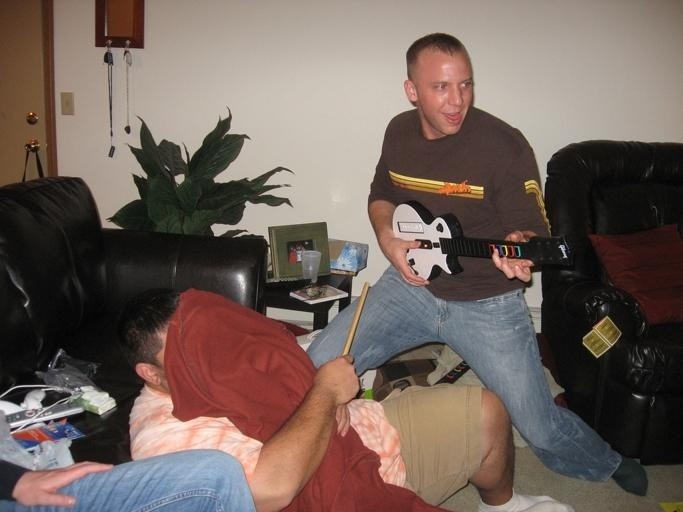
[0,177,268,467]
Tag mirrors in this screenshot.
[95,0,145,48]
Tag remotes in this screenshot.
[6,403,84,428]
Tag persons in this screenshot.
[113,284,580,512]
[298,32,651,501]
[0,446,259,512]
[288,243,305,263]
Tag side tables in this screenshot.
[266,273,352,331]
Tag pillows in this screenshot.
[588,223,683,327]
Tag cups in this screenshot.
[300,251,321,283]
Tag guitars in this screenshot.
[391,200,573,287]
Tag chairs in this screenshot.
[545,140,683,464]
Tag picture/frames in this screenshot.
[268,222,330,279]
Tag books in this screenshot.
[288,283,348,305]
